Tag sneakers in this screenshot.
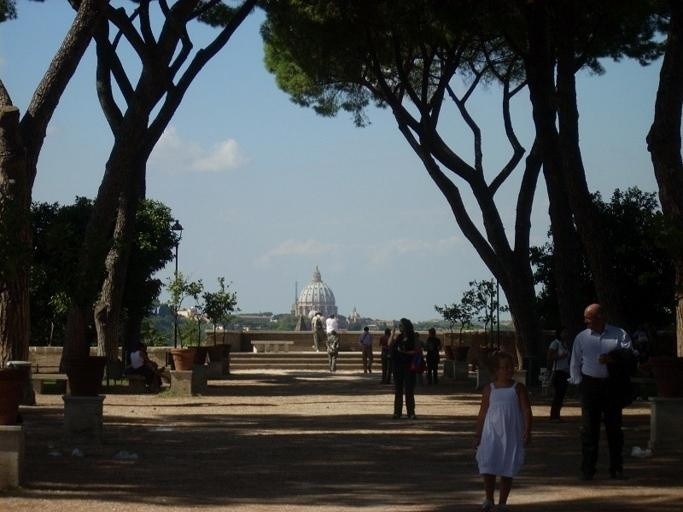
[482,498,493,509]
[496,504,510,511]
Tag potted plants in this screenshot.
[167,274,196,370]
[201,276,242,362]
[434,278,512,367]
[183,279,205,364]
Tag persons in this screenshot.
[357,326,373,374]
[325,312,338,334]
[310,311,326,351]
[544,326,571,421]
[378,326,442,385]
[389,319,419,418]
[130,341,163,393]
[469,350,533,511]
[569,302,635,476]
[326,331,340,372]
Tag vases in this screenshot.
[0,366,29,426]
[62,352,108,396]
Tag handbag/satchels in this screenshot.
[542,371,555,388]
[409,347,426,373]
